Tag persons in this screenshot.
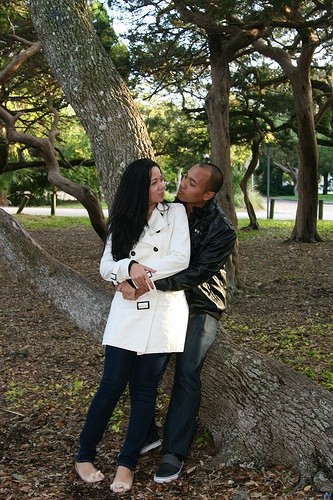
[111,162,238,484]
[73,158,191,493]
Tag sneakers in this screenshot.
[154,450,184,481]
[140,431,162,455]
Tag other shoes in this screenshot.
[75,462,105,483]
[111,471,135,492]
